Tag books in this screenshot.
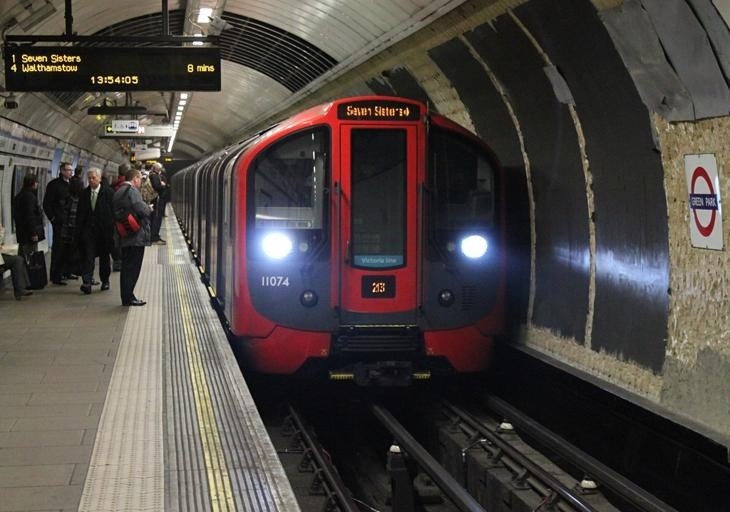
[1,243,21,256]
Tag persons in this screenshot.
[9,159,171,298]
[111,168,155,308]
[0,225,34,300]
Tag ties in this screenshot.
[91,191,97,208]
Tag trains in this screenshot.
[169,95,508,395]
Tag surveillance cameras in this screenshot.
[4,95,20,109]
[207,15,234,32]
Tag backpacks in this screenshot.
[141,175,158,201]
[54,223,84,248]
[111,185,142,238]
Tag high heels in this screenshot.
[15,291,33,301]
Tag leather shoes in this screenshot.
[100,281,109,289]
[152,239,166,245]
[92,278,99,284]
[122,299,146,306]
[80,284,91,293]
[52,281,67,285]
[66,273,78,279]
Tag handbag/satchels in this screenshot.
[24,251,48,289]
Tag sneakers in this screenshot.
[113,261,122,271]
